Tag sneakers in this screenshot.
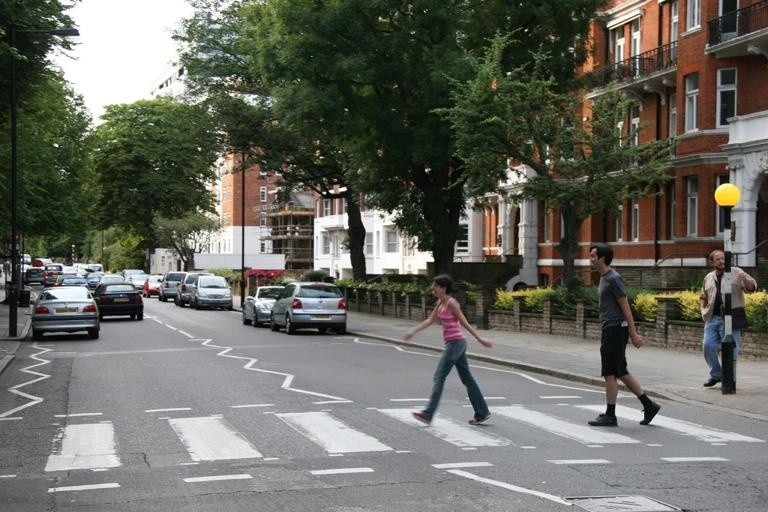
[468,414,490,424]
[413,412,430,424]
[588,414,617,426]
[640,402,660,425]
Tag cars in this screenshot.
[272,280,348,335]
[159,273,189,299]
[190,276,233,310]
[22,258,162,298]
[97,283,144,321]
[179,272,214,303]
[30,286,99,340]
[243,286,285,326]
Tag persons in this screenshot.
[587,242,661,427]
[700,249,758,387]
[403,274,493,425]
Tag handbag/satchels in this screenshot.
[722,307,748,328]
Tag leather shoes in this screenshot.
[703,378,721,387]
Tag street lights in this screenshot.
[713,181,740,395]
[7,26,79,338]
